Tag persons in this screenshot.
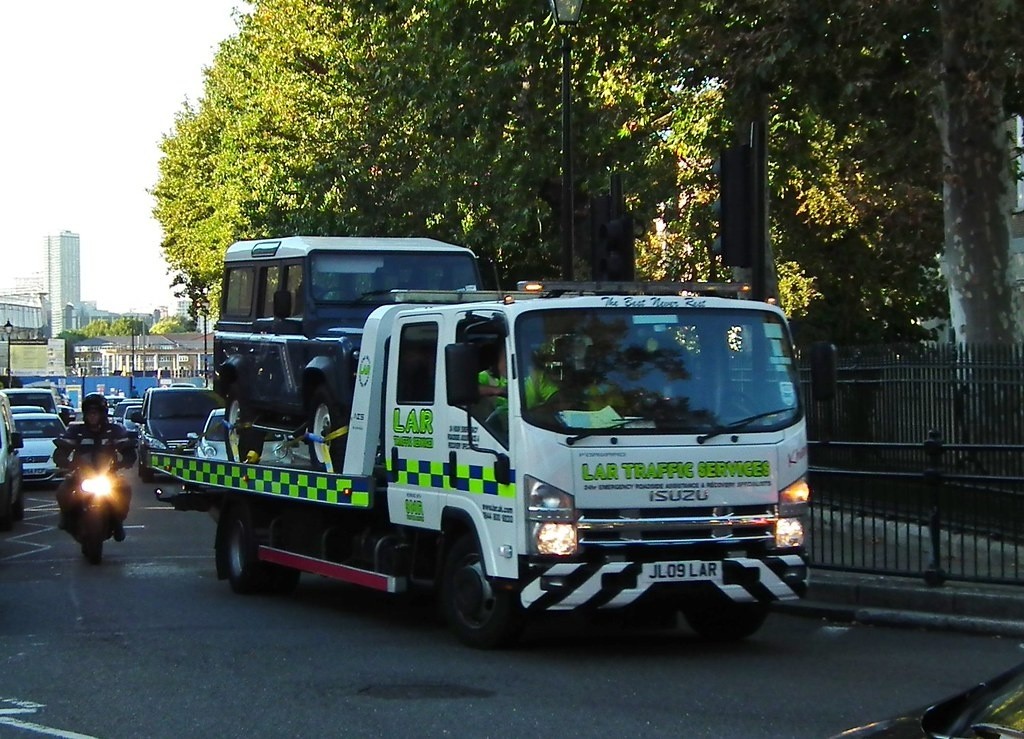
[481,331,569,440]
[53,393,138,541]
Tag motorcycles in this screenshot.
[51,435,139,565]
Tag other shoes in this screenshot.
[114,523,126,541]
[57,509,68,529]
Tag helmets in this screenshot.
[82,392,107,421]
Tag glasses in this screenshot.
[86,411,100,416]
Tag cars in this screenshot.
[1,370,225,516]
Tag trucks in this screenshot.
[149,230,844,664]
